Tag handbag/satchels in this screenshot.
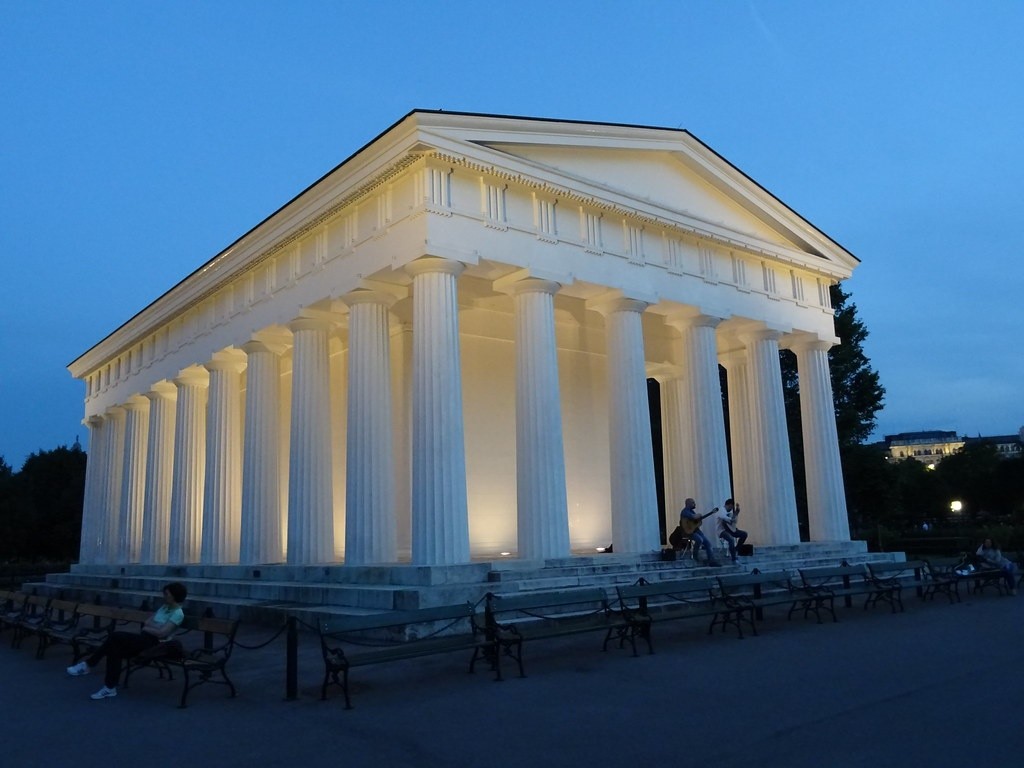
[140,639,184,661]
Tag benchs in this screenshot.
[968,552,1024,596]
[481,586,640,678]
[150,615,243,708]
[797,563,898,624]
[866,559,955,613]
[615,579,744,657]
[924,554,1004,603]
[715,570,824,637]
[52,603,118,666]
[0,592,28,649]
[72,608,154,686]
[16,595,53,660]
[36,598,77,660]
[316,603,504,710]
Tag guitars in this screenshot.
[680,507,719,535]
[723,503,740,534]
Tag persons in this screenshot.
[715,498,748,565]
[975,536,1018,596]
[67,582,187,700]
[922,521,932,533]
[680,498,722,567]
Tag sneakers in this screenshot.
[89,685,118,700]
[67,661,89,676]
[1012,589,1018,595]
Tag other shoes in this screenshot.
[694,557,700,562]
[709,562,722,567]
[735,547,738,551]
[732,556,736,561]
